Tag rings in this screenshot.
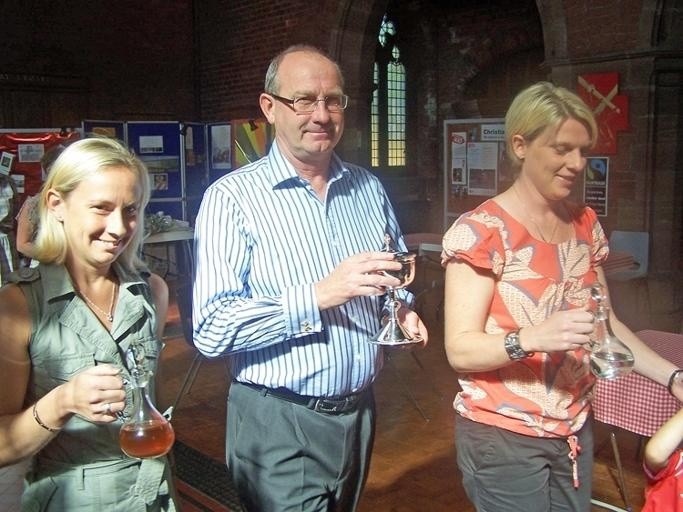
[105,403,111,414]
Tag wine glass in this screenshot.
[364,252,425,346]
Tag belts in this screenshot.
[231,377,373,414]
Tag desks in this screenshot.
[402,233,634,277]
[140,223,194,290]
[589,328,682,512]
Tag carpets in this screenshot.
[170,441,248,512]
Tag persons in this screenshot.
[0,137,184,512]
[191,44,428,512]
[441,81,683,512]
[639,408,683,512]
[15,146,63,268]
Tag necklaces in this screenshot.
[511,185,562,244]
[71,272,116,322]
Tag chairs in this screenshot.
[602,230,651,321]
[171,242,203,415]
[415,241,445,320]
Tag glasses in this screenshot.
[268,93,349,112]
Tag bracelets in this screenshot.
[503,327,536,361]
[32,397,61,434]
[667,369,681,395]
